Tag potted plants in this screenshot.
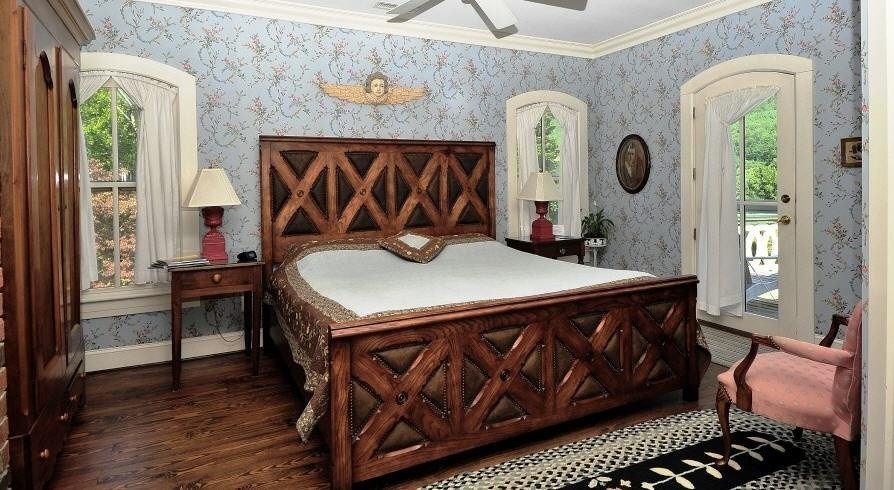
[581,209,615,246]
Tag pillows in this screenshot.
[379,230,448,263]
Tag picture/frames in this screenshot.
[616,134,651,194]
[840,137,862,167]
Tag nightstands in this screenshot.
[168,259,266,392]
[505,235,586,265]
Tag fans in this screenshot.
[386,0,519,30]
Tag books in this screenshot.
[147,254,211,269]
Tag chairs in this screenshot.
[716,300,864,490]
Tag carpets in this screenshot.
[417,404,860,490]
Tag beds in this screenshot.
[259,135,702,490]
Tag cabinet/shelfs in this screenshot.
[0,0,96,490]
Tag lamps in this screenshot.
[187,166,242,260]
[516,170,564,240]
